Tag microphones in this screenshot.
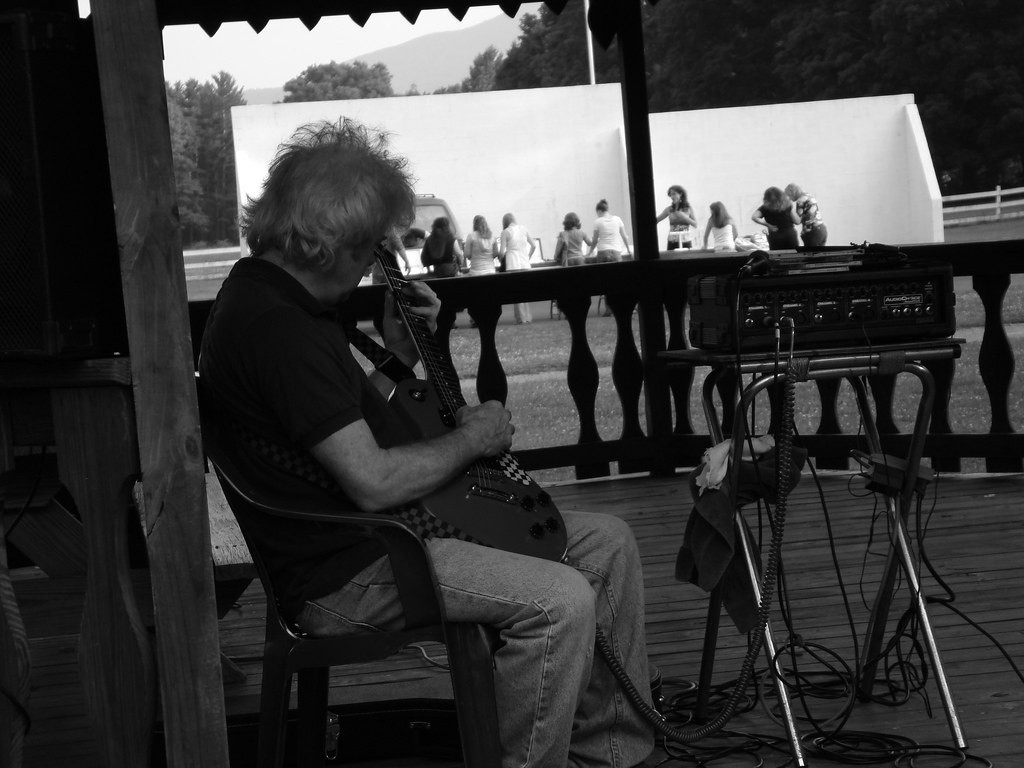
[737,253,768,277]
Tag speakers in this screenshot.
[0,8,129,359]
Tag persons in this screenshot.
[463,215,499,329]
[499,213,537,326]
[701,202,739,254]
[197,117,657,768]
[655,185,698,251]
[372,226,411,334]
[583,200,633,317]
[421,217,463,330]
[554,212,593,267]
[752,187,803,251]
[784,184,827,249]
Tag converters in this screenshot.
[863,453,935,497]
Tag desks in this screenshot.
[665,338,969,768]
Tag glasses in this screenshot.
[379,236,387,248]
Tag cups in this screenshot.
[648,664,663,707]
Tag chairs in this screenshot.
[199,319,501,768]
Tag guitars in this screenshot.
[376,249,569,564]
